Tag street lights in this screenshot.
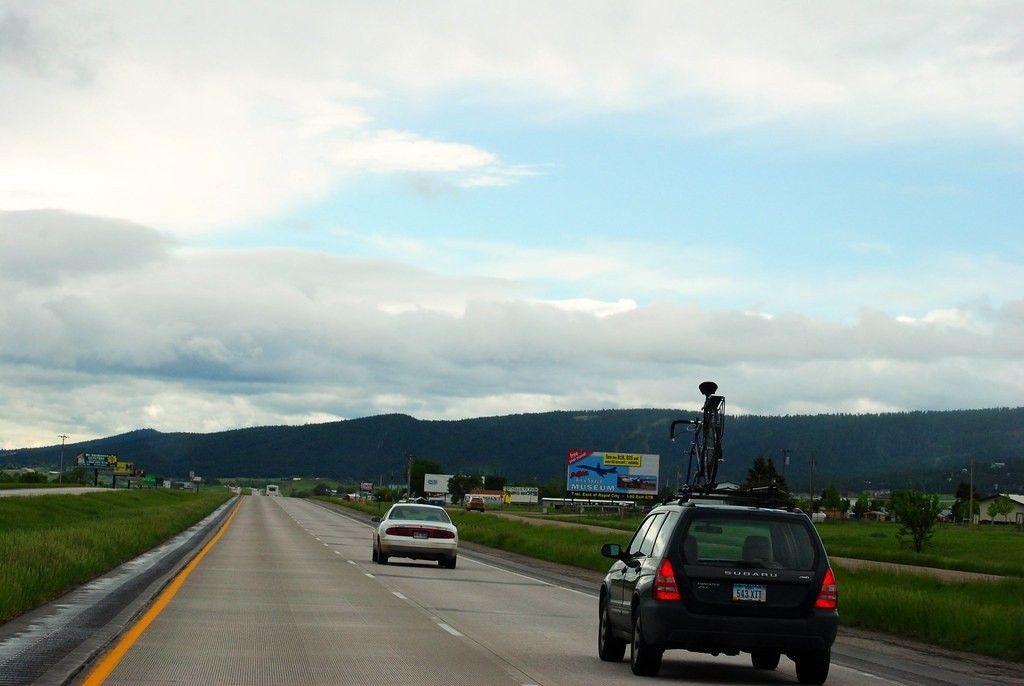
[57,434,70,484]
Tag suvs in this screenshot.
[597,488,839,684]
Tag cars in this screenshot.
[466,497,486,512]
[266,485,280,498]
[371,503,459,568]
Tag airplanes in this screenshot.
[576,461,618,479]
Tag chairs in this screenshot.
[742,536,771,563]
[426,515,442,521]
[684,534,699,563]
[395,510,406,519]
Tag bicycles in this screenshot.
[670,380,727,487]
